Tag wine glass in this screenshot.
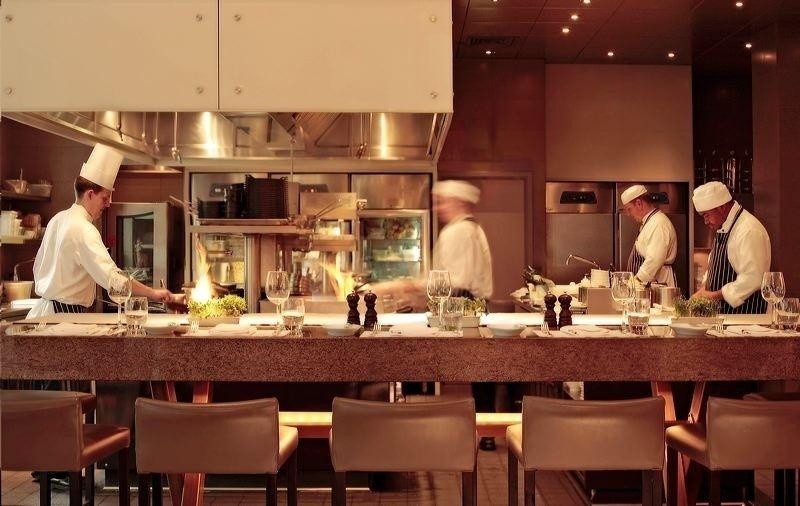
[264,270,291,328]
[426,269,452,328]
[760,271,786,330]
[609,269,637,333]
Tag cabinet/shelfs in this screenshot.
[358,209,430,280]
[0,188,50,245]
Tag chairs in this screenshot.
[506,395,665,505]
[1,398,132,506]
[134,398,299,506]
[328,396,478,506]
[666,397,799,506]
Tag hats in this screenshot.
[621,185,647,205]
[692,181,733,213]
[80,143,123,192]
[432,181,480,204]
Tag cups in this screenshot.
[440,295,463,333]
[281,297,306,336]
[626,287,651,334]
[107,270,133,331]
[777,297,800,331]
[124,293,149,337]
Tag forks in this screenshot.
[370,321,382,336]
[540,320,554,336]
[715,322,724,333]
[38,320,48,330]
[188,320,200,333]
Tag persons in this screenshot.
[621,184,678,308]
[690,181,771,314]
[377,181,493,315]
[32,142,175,490]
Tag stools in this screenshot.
[0,389,97,506]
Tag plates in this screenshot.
[669,322,709,336]
[487,322,527,337]
[142,320,181,334]
[322,321,363,337]
